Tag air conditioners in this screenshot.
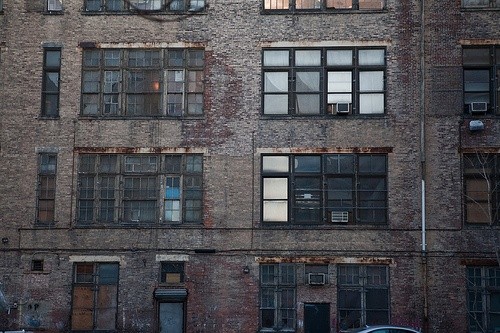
[471,102,488,113]
[337,103,351,114]
[331,211,350,223]
[308,273,326,286]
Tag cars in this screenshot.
[340,325,419,333]
